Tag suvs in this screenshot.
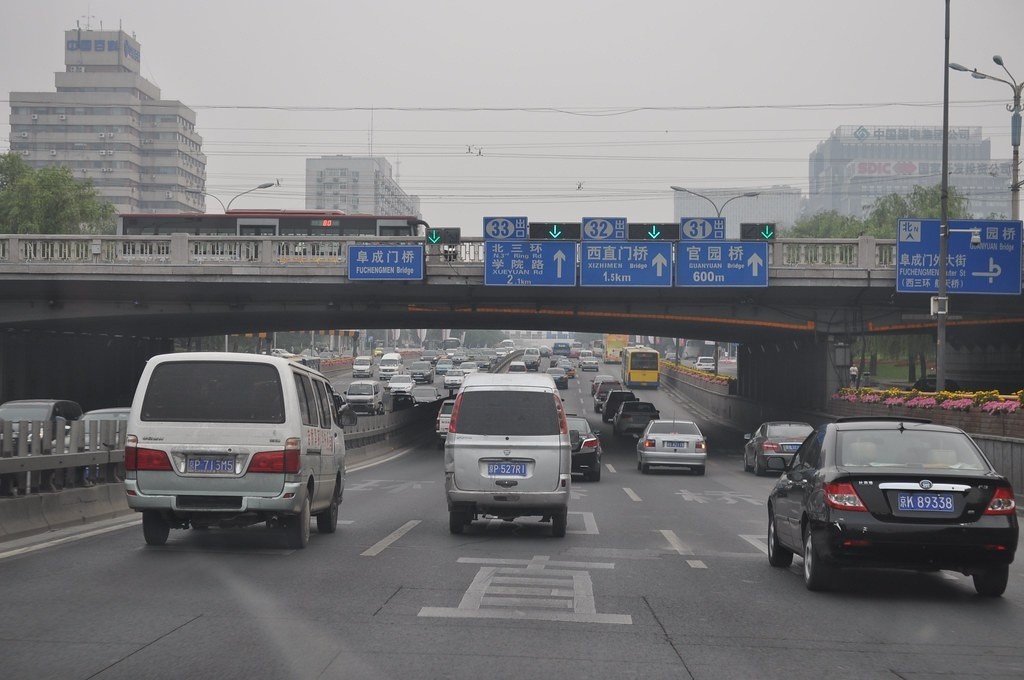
[602,389,640,423]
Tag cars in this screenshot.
[566,415,603,482]
[633,420,707,476]
[767,415,1019,598]
[743,421,815,476]
[0,400,131,453]
[612,400,660,438]
[589,374,622,413]
[386,339,602,450]
[666,352,716,373]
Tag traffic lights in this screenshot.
[426,228,460,245]
[529,222,582,241]
[628,223,680,242]
[740,223,775,240]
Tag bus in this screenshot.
[441,337,461,357]
[117,209,430,263]
[601,334,629,364]
[619,345,660,389]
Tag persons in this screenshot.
[850,363,858,384]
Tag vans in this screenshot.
[352,352,404,380]
[443,372,580,537]
[124,351,358,548]
[344,380,383,415]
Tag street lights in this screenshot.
[949,55,1023,221]
[671,186,761,375]
[184,183,276,351]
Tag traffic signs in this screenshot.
[678,242,770,289]
[896,219,1022,295]
[580,241,673,288]
[485,240,577,288]
